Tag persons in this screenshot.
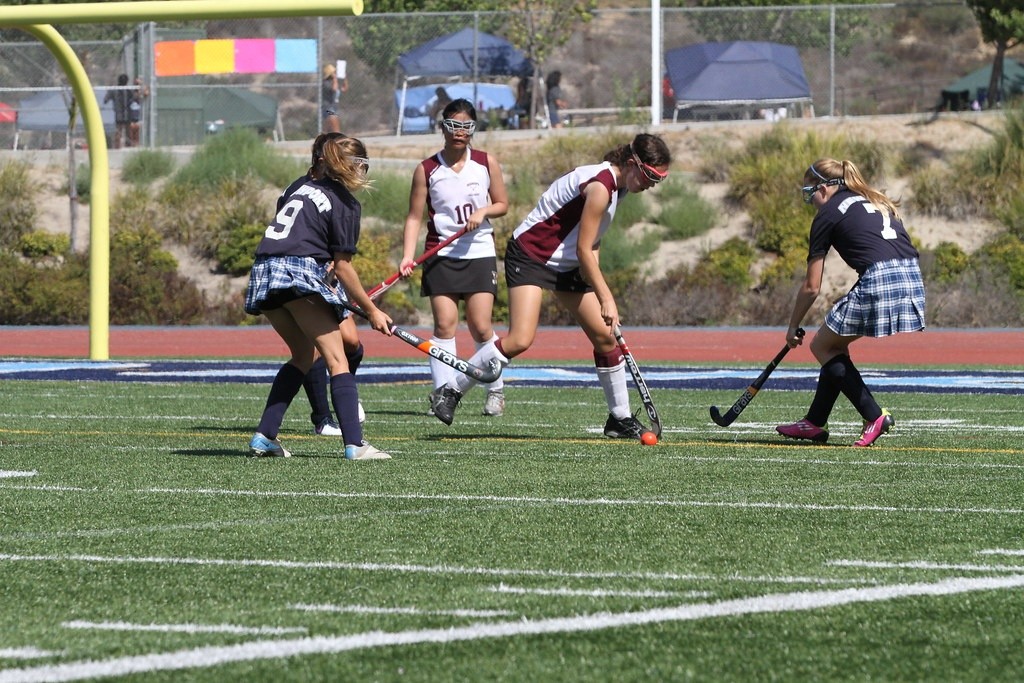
[775,159,926,447]
[321,65,347,134]
[398,99,509,417]
[428,133,673,438]
[104,75,150,151]
[426,70,568,127]
[243,133,395,460]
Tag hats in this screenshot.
[323,64,336,80]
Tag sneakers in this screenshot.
[776,418,829,443]
[604,407,651,440]
[345,440,392,460]
[854,408,895,447]
[427,383,464,426]
[482,388,504,416]
[315,417,342,435]
[358,402,365,423]
[249,432,292,458]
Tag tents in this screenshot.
[664,40,811,122]
[940,57,1024,112]
[0,87,282,143]
[394,27,536,124]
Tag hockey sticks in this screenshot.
[592,285,664,439]
[708,328,806,428]
[352,226,468,310]
[338,297,503,384]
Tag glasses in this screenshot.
[630,140,667,183]
[443,119,476,135]
[344,156,369,173]
[802,178,845,203]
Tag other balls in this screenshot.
[641,432,658,446]
